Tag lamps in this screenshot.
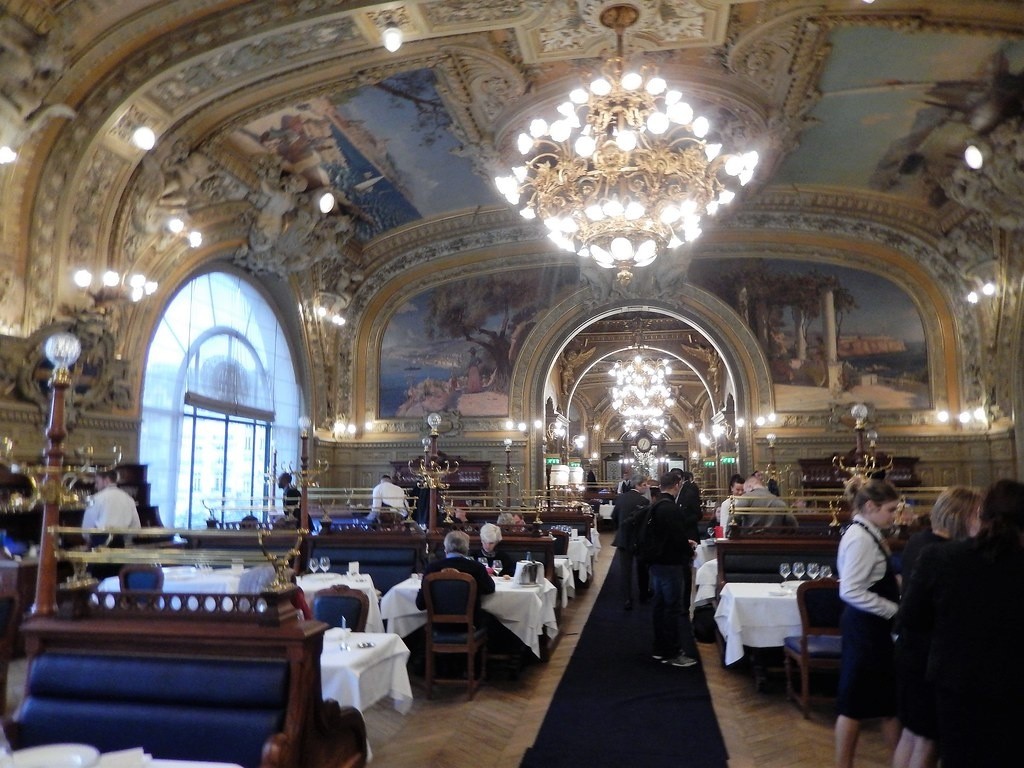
[964,140,994,170]
[608,318,676,439]
[503,438,512,450]
[298,415,311,436]
[422,437,431,450]
[867,430,878,446]
[44,332,81,380]
[851,404,868,427]
[428,413,441,433]
[493,4,759,288]
[380,16,403,53]
[767,433,776,446]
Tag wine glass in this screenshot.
[466,557,503,580]
[551,525,572,537]
[780,562,832,588]
[708,527,714,538]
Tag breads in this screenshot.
[503,574,510,580]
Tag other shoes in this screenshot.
[653,650,685,660]
[639,588,653,600]
[625,600,633,610]
[663,656,696,667]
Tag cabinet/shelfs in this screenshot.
[0,464,175,546]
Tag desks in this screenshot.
[599,504,616,520]
[0,557,74,658]
[567,536,594,583]
[380,577,559,688]
[554,555,575,609]
[693,558,719,603]
[713,582,803,666]
[590,528,602,562]
[92,566,385,633]
[694,538,728,567]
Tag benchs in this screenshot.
[0,494,932,768]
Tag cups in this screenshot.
[309,557,331,575]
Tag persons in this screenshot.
[413,530,496,635]
[242,516,258,530]
[367,474,411,530]
[702,470,799,539]
[278,472,315,532]
[497,509,527,533]
[831,478,1024,768]
[469,521,516,578]
[78,471,141,579]
[440,502,472,535]
[608,466,703,667]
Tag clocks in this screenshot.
[636,436,652,453]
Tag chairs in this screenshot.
[424,568,486,700]
[312,585,370,632]
[119,563,164,607]
[784,581,846,719]
